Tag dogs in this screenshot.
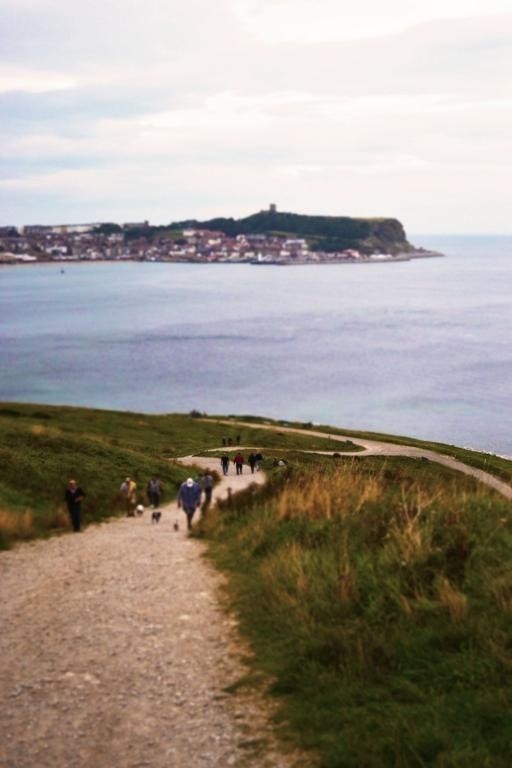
[152,512,161,525]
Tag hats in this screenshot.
[186,478,192,487]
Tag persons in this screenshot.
[119,477,137,516]
[218,434,288,476]
[149,474,166,508]
[176,476,202,530]
[197,471,215,507]
[63,478,85,532]
